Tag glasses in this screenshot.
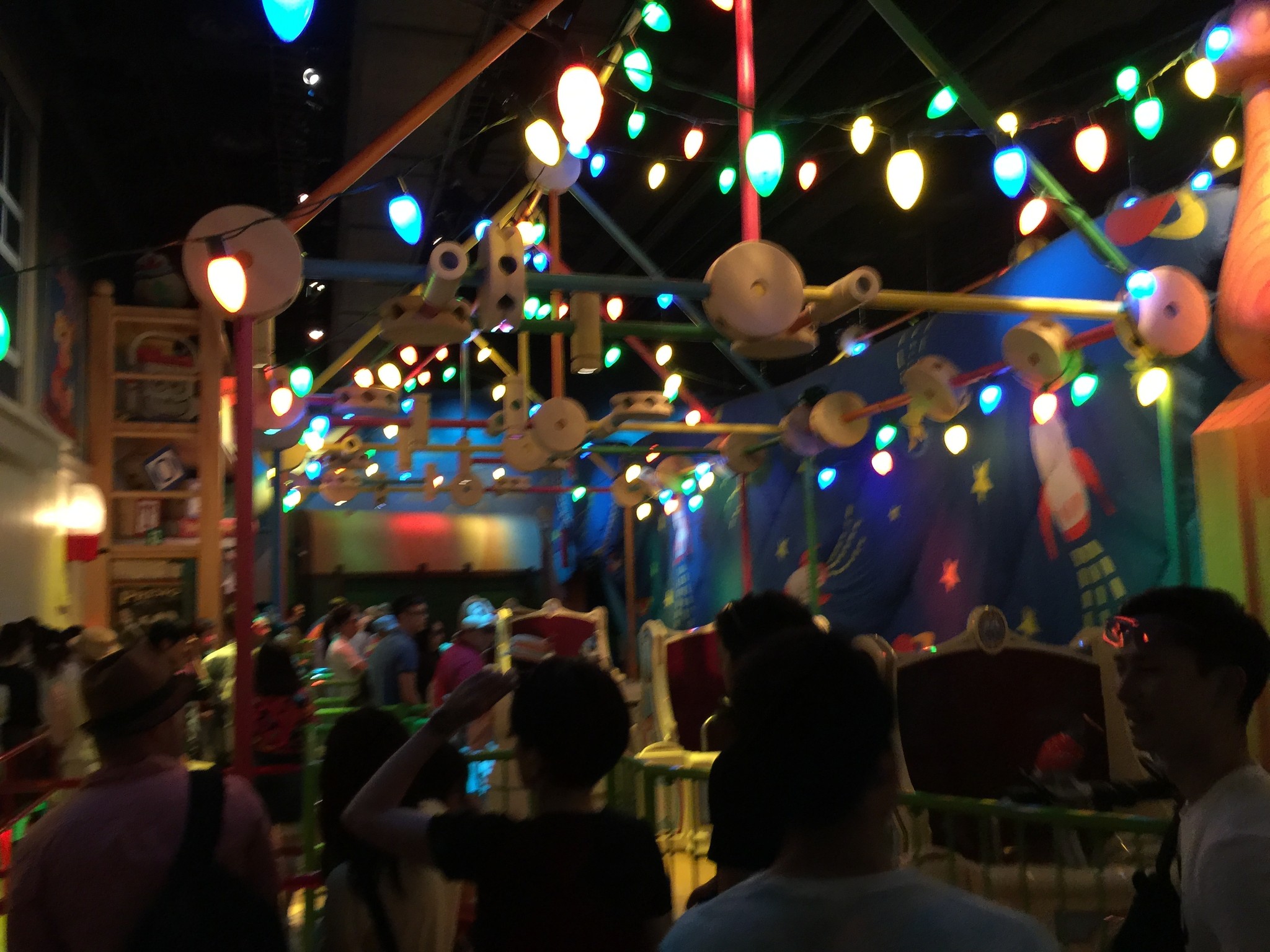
[404,609,428,617]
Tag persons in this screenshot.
[0,572,671,822]
[317,701,465,952]
[248,642,315,933]
[340,654,673,952]
[706,587,826,897]
[656,629,1064,952]
[1110,586,1270,952]
[7,645,281,952]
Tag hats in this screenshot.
[1,617,36,658]
[80,650,198,737]
[67,626,122,658]
[332,605,357,626]
[272,620,291,636]
[149,619,198,651]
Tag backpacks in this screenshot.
[1107,815,1185,951]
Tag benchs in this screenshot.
[853,603,1172,917]
[636,614,829,859]
[494,596,625,814]
